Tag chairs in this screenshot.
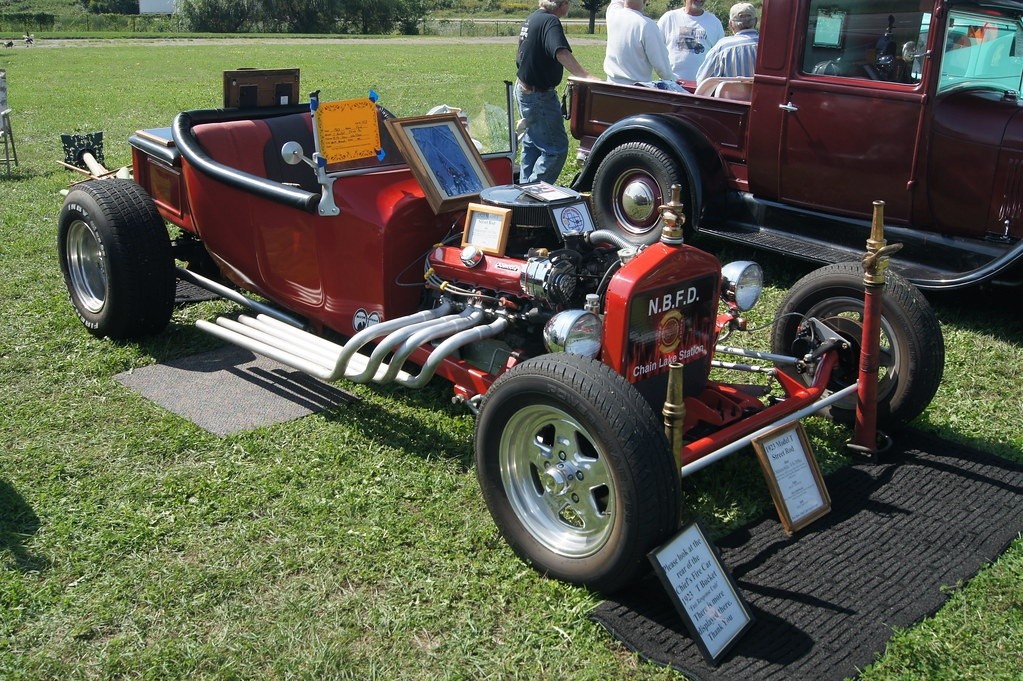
[813,56,913,87]
[0,69,19,177]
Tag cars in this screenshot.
[57,68,950,590]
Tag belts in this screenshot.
[517,77,556,93]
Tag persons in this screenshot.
[513,1,601,182]
[436,163,473,197]
[656,2,726,81]
[604,0,684,87]
[695,1,762,87]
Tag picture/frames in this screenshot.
[643,519,753,665]
[460,202,514,259]
[383,113,497,215]
[516,179,577,203]
[547,199,598,242]
[750,418,834,537]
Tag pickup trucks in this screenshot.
[568,0,1023,297]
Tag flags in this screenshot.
[432,151,451,176]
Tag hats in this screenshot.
[730,2,757,20]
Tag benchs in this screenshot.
[190,108,312,187]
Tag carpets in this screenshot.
[111,343,365,440]
[587,429,1023,679]
